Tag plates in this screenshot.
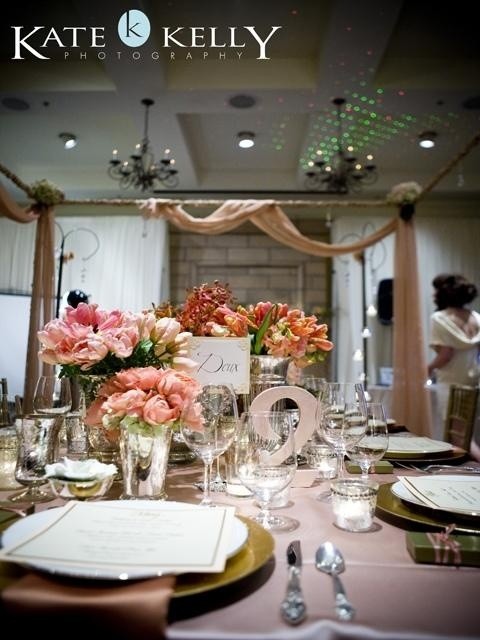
[1,500,278,602]
[381,443,469,464]
[370,481,479,534]
[391,474,479,519]
[356,437,453,456]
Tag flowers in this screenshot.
[386,180,422,203]
[33,303,201,377]
[204,296,335,369]
[27,177,66,209]
[80,366,208,440]
[144,278,246,338]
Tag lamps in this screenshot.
[338,221,388,406]
[108,95,187,196]
[303,96,380,205]
[236,130,254,149]
[419,129,435,148]
[60,129,78,146]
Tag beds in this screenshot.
[0,409,478,639]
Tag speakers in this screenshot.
[376,277,394,326]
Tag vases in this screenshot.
[241,354,294,424]
[400,205,418,220]
[113,419,175,503]
[61,369,114,463]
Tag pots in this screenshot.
[315,542,355,620]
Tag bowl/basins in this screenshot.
[46,477,114,497]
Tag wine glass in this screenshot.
[179,379,389,530]
[12,375,88,501]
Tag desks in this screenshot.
[368,384,444,441]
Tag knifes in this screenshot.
[281,540,308,625]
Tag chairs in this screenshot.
[0,377,8,418]
[13,395,25,413]
[441,383,479,452]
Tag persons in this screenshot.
[426,274,480,387]
